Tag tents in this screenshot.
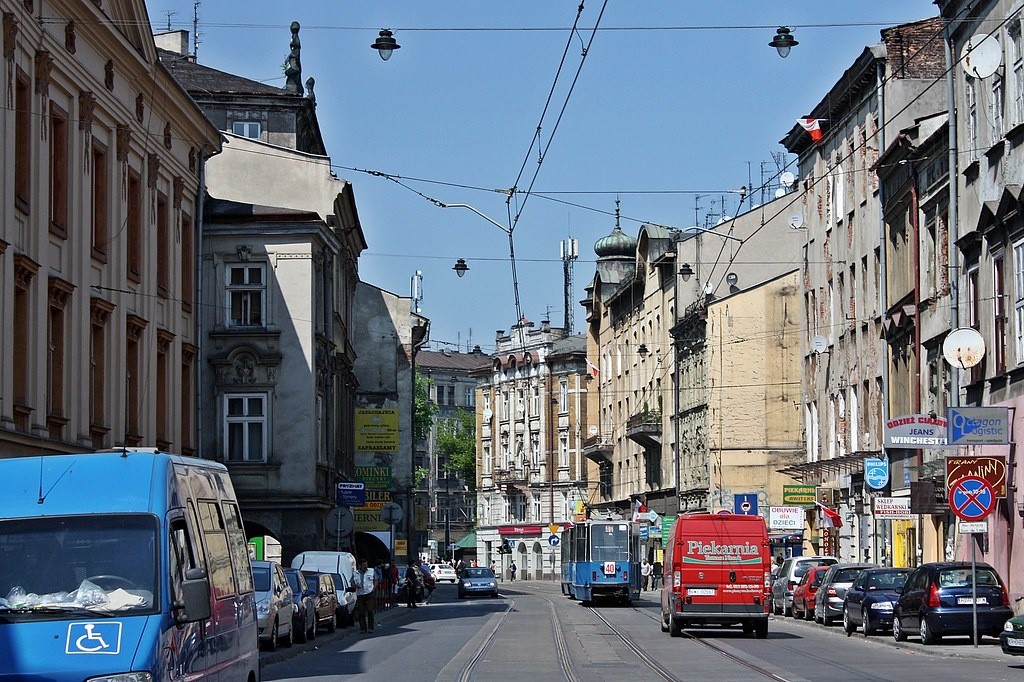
[455,534,478,547]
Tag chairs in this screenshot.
[966,575,972,583]
[838,573,849,581]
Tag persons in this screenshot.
[489,560,496,575]
[374,560,437,611]
[420,555,477,579]
[641,556,660,593]
[510,560,517,584]
[14,534,150,596]
[771,553,784,583]
[350,559,378,634]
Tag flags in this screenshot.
[564,521,572,532]
[587,361,602,377]
[633,500,647,522]
[797,118,825,146]
[820,505,843,528]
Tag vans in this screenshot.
[653,514,771,639]
[0,436,260,682]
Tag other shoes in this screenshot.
[413,605,419,608]
[368,629,374,633]
[408,605,412,608]
[360,629,366,634]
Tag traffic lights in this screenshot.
[502,538,509,553]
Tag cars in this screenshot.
[250,551,436,650]
[457,567,499,599]
[892,561,1014,645]
[770,556,917,636]
[999,613,1024,656]
[428,564,457,583]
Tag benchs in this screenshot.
[870,578,904,588]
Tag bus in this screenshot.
[560,508,641,606]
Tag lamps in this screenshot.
[636,343,649,358]
[371,30,401,60]
[676,261,696,282]
[583,373,594,384]
[453,259,470,276]
[768,27,800,60]
